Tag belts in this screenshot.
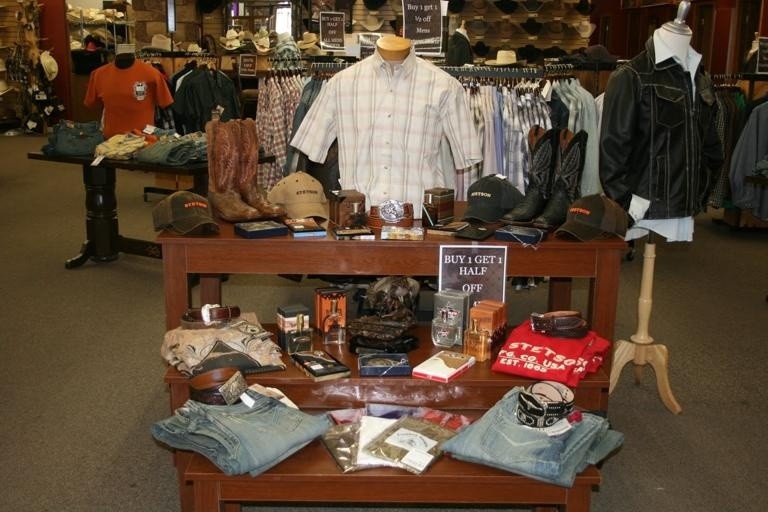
[180,314,225,330]
[529,310,588,339]
[187,367,246,405]
[179,300,240,321]
[516,379,575,428]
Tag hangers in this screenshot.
[439,65,542,97]
[263,54,304,86]
[309,61,357,83]
[182,51,219,79]
[710,72,745,98]
[544,63,580,89]
[136,51,162,67]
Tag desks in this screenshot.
[154,198,629,344]
[26,147,233,287]
[183,411,601,512]
[163,323,610,467]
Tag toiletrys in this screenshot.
[288,315,312,354]
[345,203,363,228]
[422,195,438,227]
[322,299,346,345]
[431,310,456,350]
[463,320,489,362]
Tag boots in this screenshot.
[532,127,588,230]
[230,117,287,219]
[498,124,561,225]
[204,120,261,222]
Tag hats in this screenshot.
[494,0,519,14]
[472,41,489,57]
[461,173,524,225]
[572,20,597,39]
[520,18,542,34]
[266,171,328,221]
[446,0,465,13]
[542,20,568,40]
[546,0,573,18]
[465,18,489,36]
[470,0,489,15]
[484,47,527,67]
[40,50,58,82]
[519,0,544,13]
[218,27,272,55]
[358,14,385,32]
[67,6,95,19]
[585,43,620,63]
[544,46,566,59]
[490,18,517,37]
[0,80,12,97]
[575,0,595,15]
[142,33,176,52]
[518,44,540,62]
[152,190,221,236]
[297,31,320,49]
[553,194,628,243]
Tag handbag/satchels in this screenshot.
[5,43,29,84]
[24,71,65,133]
